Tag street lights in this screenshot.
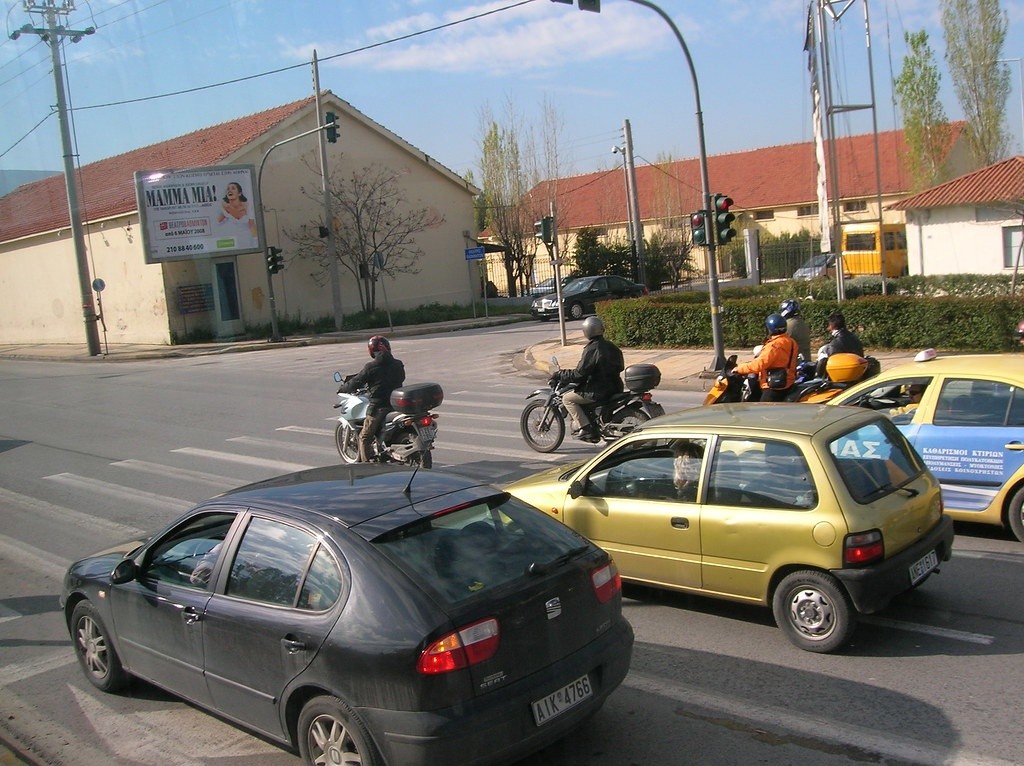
[612,145,639,282]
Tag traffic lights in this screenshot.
[266,245,285,275]
[690,210,707,245]
[325,112,342,144]
[714,194,738,246]
[533,217,553,244]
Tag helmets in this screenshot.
[582,316,604,340]
[765,314,788,336]
[780,299,799,319]
[367,335,391,359]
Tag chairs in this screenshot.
[456,522,496,560]
[949,383,1010,424]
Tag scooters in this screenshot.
[701,339,880,411]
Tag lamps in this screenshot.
[58,229,70,236]
[100,219,116,228]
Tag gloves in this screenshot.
[548,371,561,385]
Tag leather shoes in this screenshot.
[575,426,593,439]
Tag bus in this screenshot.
[842,221,910,281]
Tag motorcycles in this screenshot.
[333,371,443,470]
[518,354,667,455]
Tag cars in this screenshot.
[531,274,648,321]
[522,274,568,300]
[57,461,637,766]
[717,348,1024,546]
[423,400,958,656]
[793,252,838,282]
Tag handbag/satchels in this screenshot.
[767,367,786,388]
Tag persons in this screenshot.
[548,317,625,440]
[731,315,798,402]
[190,539,225,586]
[593,282,599,288]
[816,311,864,379]
[889,384,925,420]
[672,443,704,501]
[218,182,257,237]
[337,336,405,461]
[780,299,811,363]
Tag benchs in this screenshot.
[754,458,881,505]
[715,441,798,507]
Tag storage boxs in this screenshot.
[390,383,443,413]
[625,364,662,390]
[825,353,868,382]
